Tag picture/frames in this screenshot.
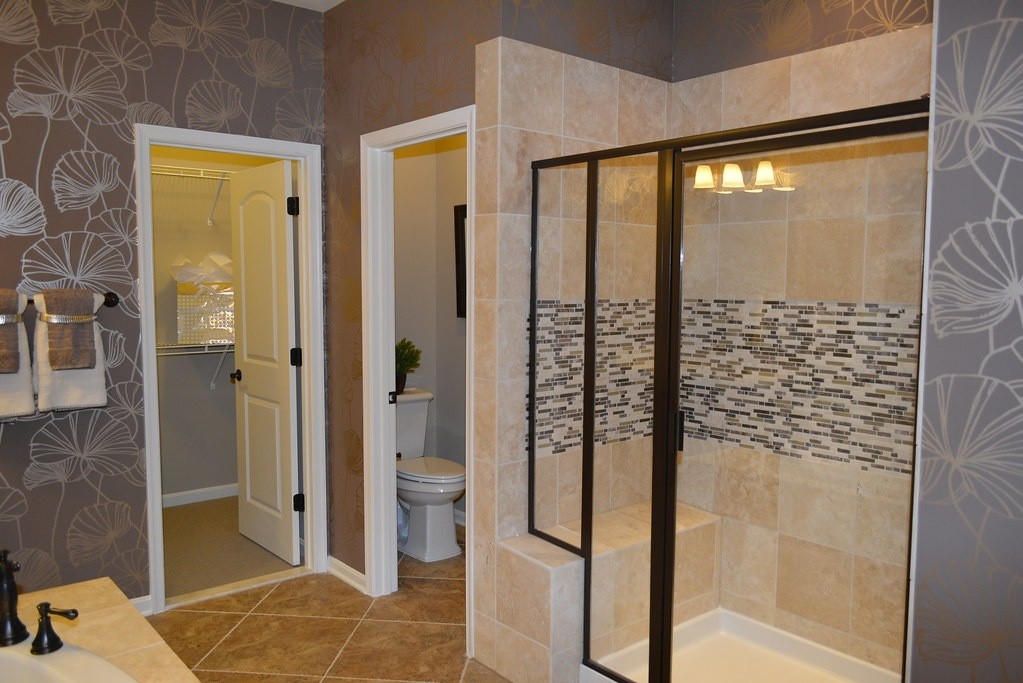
[453,204,466,317]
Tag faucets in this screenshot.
[0,550,30,649]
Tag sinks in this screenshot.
[0,577,201,683]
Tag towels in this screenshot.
[0,288,108,423]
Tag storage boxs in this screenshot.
[176,281,236,347]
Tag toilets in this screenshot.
[395,387,465,563]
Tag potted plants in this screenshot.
[395,337,421,395]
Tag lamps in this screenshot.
[721,161,745,188]
[755,158,775,185]
[693,164,714,189]
